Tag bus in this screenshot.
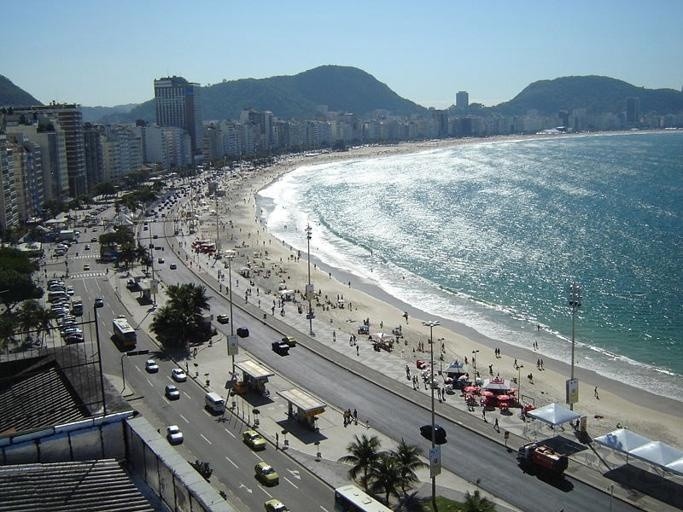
[112,317,137,346]
[335,484,390,511]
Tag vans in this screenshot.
[204,392,225,413]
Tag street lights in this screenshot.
[304,223,315,334]
[472,350,479,384]
[567,279,583,411]
[423,320,441,497]
[438,337,445,373]
[516,364,524,400]
[213,200,220,255]
[221,248,237,373]
[145,217,160,310]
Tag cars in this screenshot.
[47,279,84,344]
[217,314,229,324]
[237,327,249,337]
[170,263,177,269]
[265,498,288,512]
[272,335,296,353]
[171,368,186,382]
[84,264,89,270]
[158,257,164,262]
[165,424,184,444]
[145,358,159,374]
[54,225,97,256]
[254,461,280,485]
[243,429,266,449]
[81,204,111,223]
[419,424,447,440]
[95,297,104,307]
[166,384,179,399]
[149,243,154,248]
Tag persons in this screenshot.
[171,164,544,431]
[276,433,279,443]
[593,386,599,399]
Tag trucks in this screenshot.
[518,442,568,474]
